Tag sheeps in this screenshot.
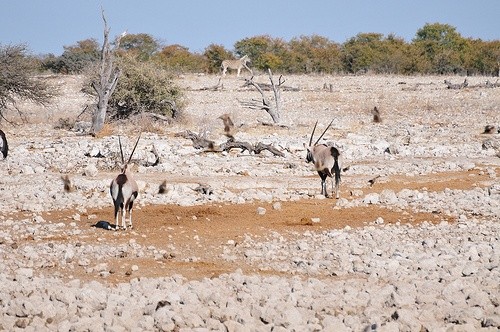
[110,137,139,231]
[302,118,341,199]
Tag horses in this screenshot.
[221,54,253,76]
